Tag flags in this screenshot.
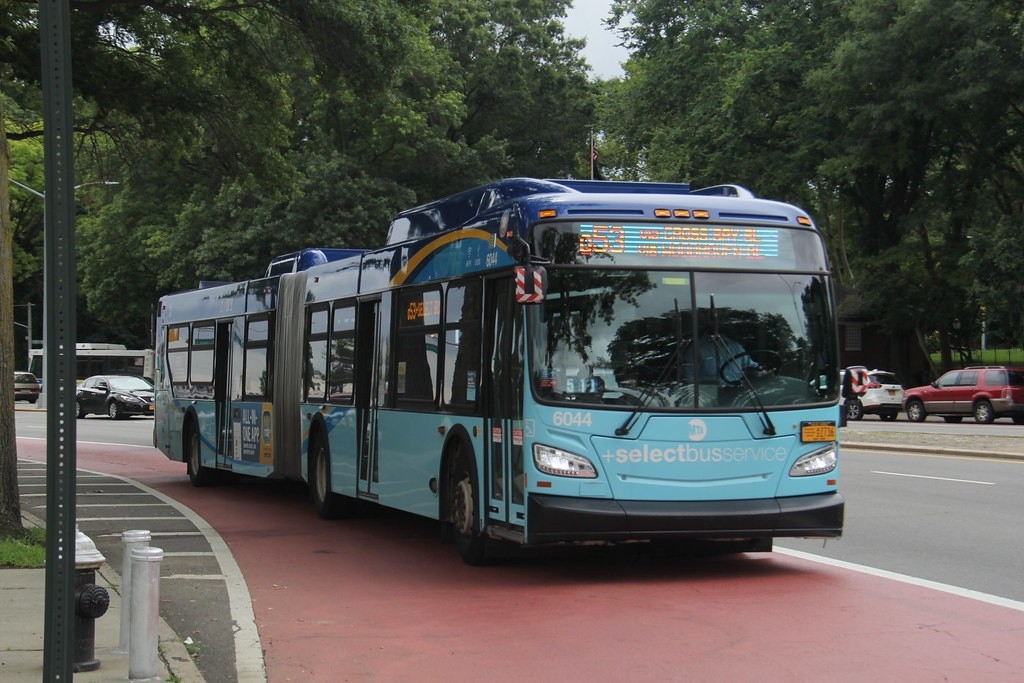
[589,139,598,161]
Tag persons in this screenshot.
[678,304,767,387]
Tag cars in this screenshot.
[75,374,156,419]
[14,370,41,404]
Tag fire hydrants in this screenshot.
[71,523,111,674]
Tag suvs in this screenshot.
[903,365,1024,426]
[808,366,905,422]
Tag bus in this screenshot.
[152,176,848,570]
